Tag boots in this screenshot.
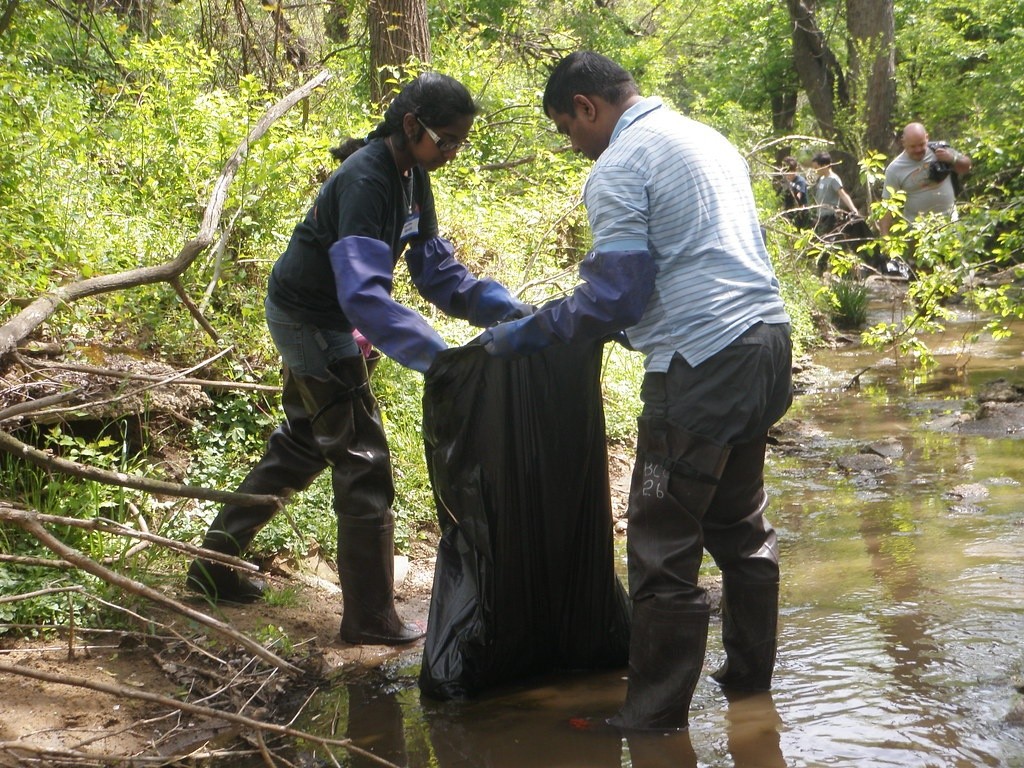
[336,515,429,646]
[710,580,780,695]
[582,602,710,736]
[185,485,293,607]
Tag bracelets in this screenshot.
[950,155,958,167]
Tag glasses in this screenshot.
[405,105,470,153]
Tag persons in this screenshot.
[187,72,538,644]
[483,52,794,734]
[782,156,807,228]
[879,124,971,318]
[812,153,860,277]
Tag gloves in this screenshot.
[404,236,528,327]
[323,233,452,376]
[482,235,658,360]
